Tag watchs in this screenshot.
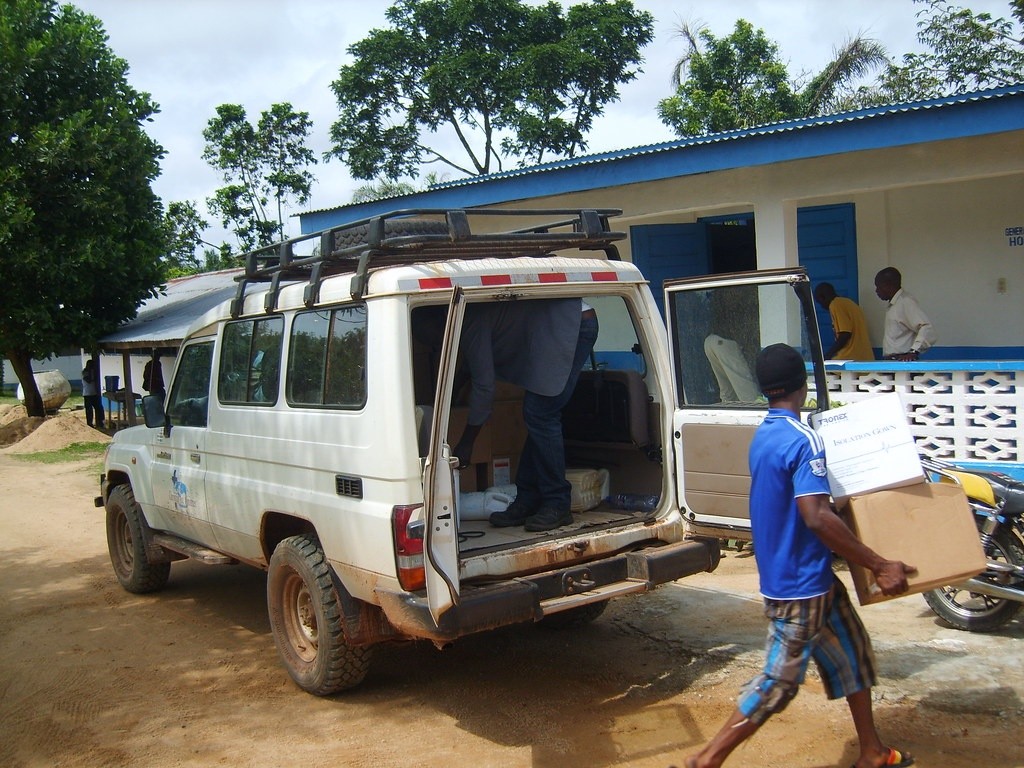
[909,349,919,354]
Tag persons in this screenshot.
[704,288,761,401]
[142,349,166,406]
[814,282,875,362]
[428,298,600,531]
[874,267,936,361]
[82,360,106,429]
[672,344,917,768]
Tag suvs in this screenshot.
[91,208,832,701]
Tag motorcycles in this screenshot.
[917,451,1024,632]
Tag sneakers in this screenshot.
[489,487,541,526]
[524,494,574,531]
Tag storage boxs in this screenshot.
[435,373,528,494]
[808,401,923,503]
[838,479,987,605]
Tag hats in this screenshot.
[756,343,807,401]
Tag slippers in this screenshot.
[850,747,913,768]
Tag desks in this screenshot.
[103,391,141,430]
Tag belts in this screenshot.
[582,308,597,321]
